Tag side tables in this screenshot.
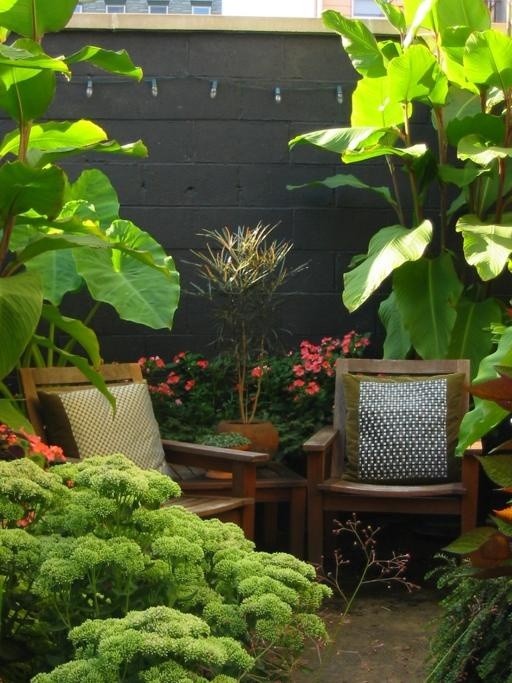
[171,462,308,563]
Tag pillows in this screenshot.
[35,380,185,484]
[340,369,466,486]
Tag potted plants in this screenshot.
[179,219,313,466]
[190,428,253,481]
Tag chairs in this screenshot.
[18,361,270,543]
[300,358,483,577]
